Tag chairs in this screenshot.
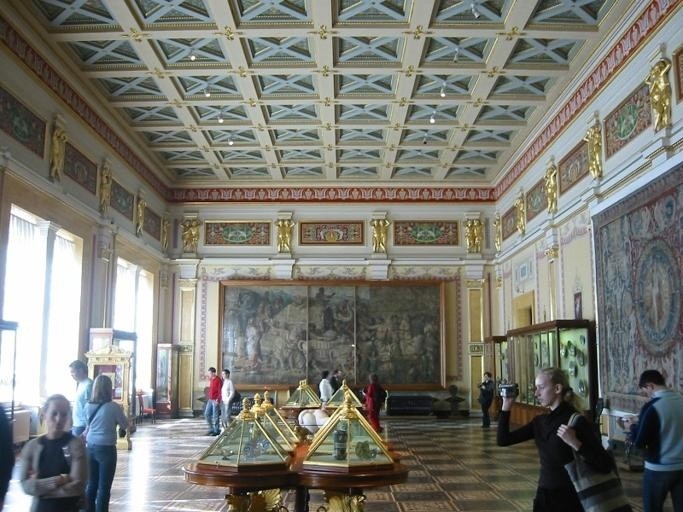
[138,391,156,425]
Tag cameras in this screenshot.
[499,383,520,397]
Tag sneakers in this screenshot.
[481,424,489,428]
[207,431,220,436]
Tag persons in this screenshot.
[317,369,333,403]
[495,366,614,511]
[80,374,130,511]
[622,369,683,511]
[274,219,285,253]
[218,368,235,429]
[378,220,391,253]
[67,360,94,443]
[368,219,380,253]
[490,56,673,255]
[232,286,441,384]
[330,369,342,395]
[361,373,383,433]
[476,372,493,428]
[463,220,474,253]
[204,366,222,436]
[19,394,86,512]
[473,219,485,254]
[282,220,295,253]
[49,127,202,258]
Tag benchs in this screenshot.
[231,397,273,415]
[389,396,434,416]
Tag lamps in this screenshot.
[187,54,234,147]
[422,0,481,146]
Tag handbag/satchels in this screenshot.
[564,450,633,512]
[232,390,241,402]
